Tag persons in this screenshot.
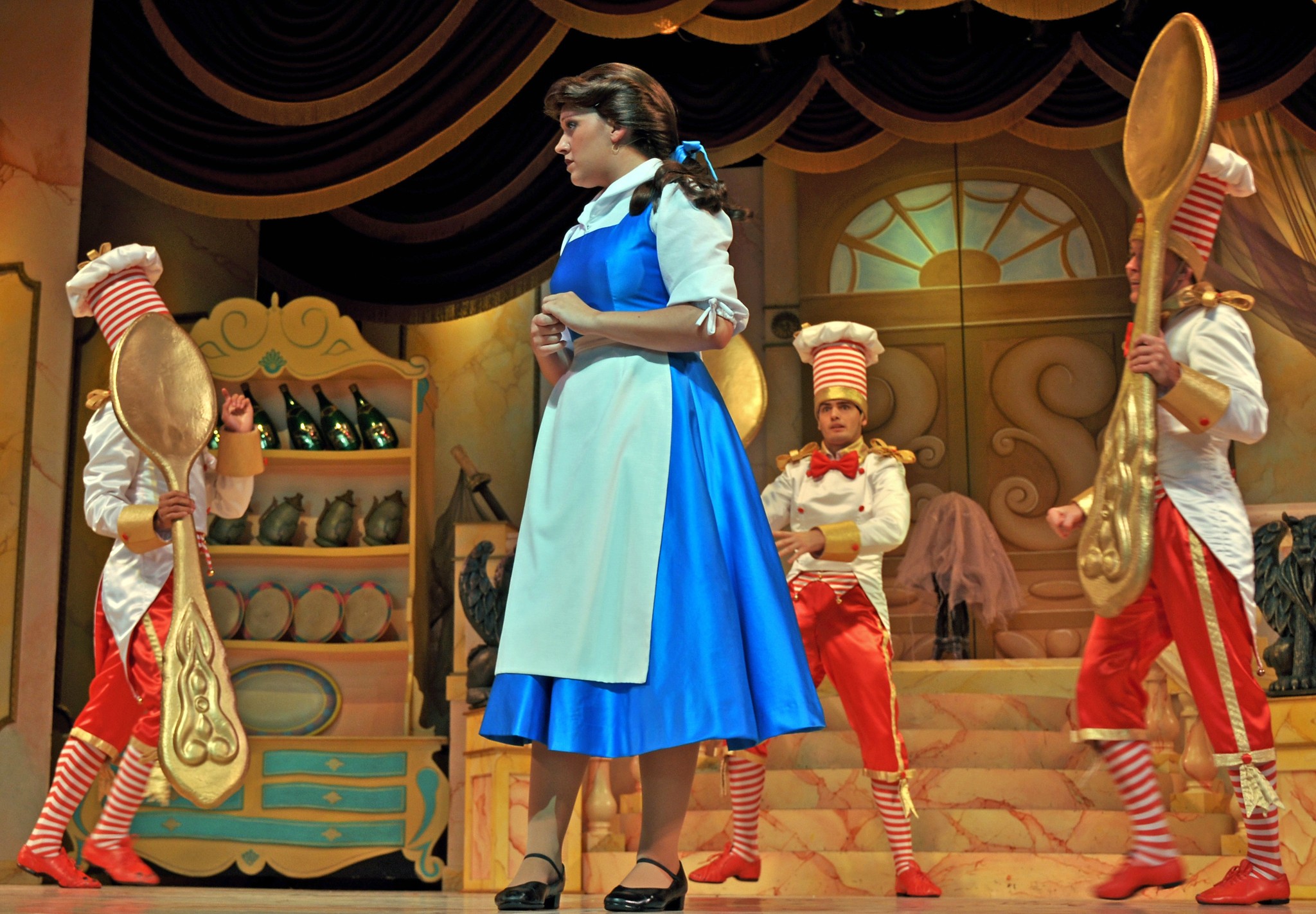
[687,322,941,901]
[19,235,265,887]
[481,57,824,914]
[1048,143,1299,907]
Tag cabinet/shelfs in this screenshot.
[45,291,452,892]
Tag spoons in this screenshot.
[1079,10,1217,619]
[113,314,251,808]
[699,331,767,449]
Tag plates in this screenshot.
[290,581,344,642]
[340,579,392,641]
[203,580,245,639]
[241,581,294,641]
[228,659,343,737]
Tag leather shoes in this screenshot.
[604,857,689,912]
[1093,852,1185,903]
[493,853,567,910]
[80,832,162,886]
[16,843,103,888]
[1195,865,1292,907]
[689,842,761,884]
[894,868,942,898]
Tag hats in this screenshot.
[790,320,886,417]
[64,237,175,359]
[1128,138,1257,285]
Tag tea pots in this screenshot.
[205,504,253,545]
[364,491,407,545]
[314,489,357,548]
[257,492,304,544]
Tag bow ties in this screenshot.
[808,451,861,480]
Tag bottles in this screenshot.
[241,381,281,449]
[311,382,362,451]
[209,410,224,449]
[350,383,398,450]
[279,382,327,449]
